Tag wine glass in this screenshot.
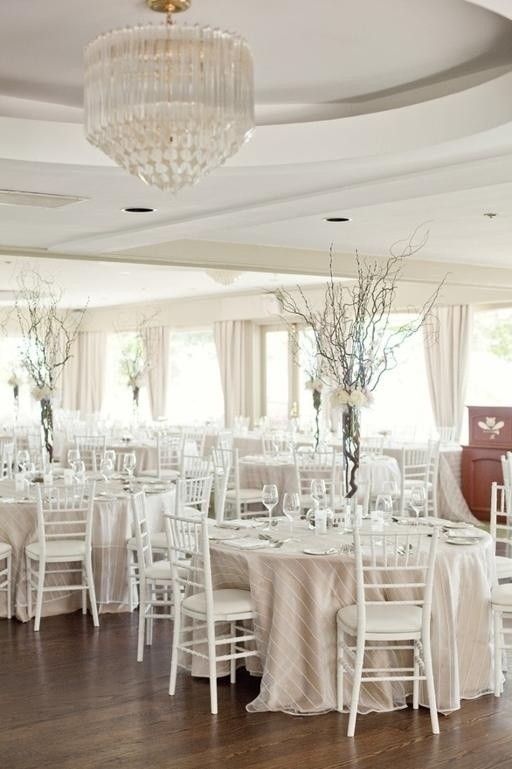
[13,445,170,503]
[208,479,485,553]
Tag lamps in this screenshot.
[83,0,257,195]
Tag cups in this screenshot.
[234,416,250,434]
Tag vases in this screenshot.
[344,405,361,533]
[311,390,323,454]
[132,387,139,437]
[41,400,53,493]
[14,385,19,430]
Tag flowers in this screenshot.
[260,220,447,497]
[0,264,90,463]
[113,309,160,405]
[277,315,324,453]
[8,372,24,397]
